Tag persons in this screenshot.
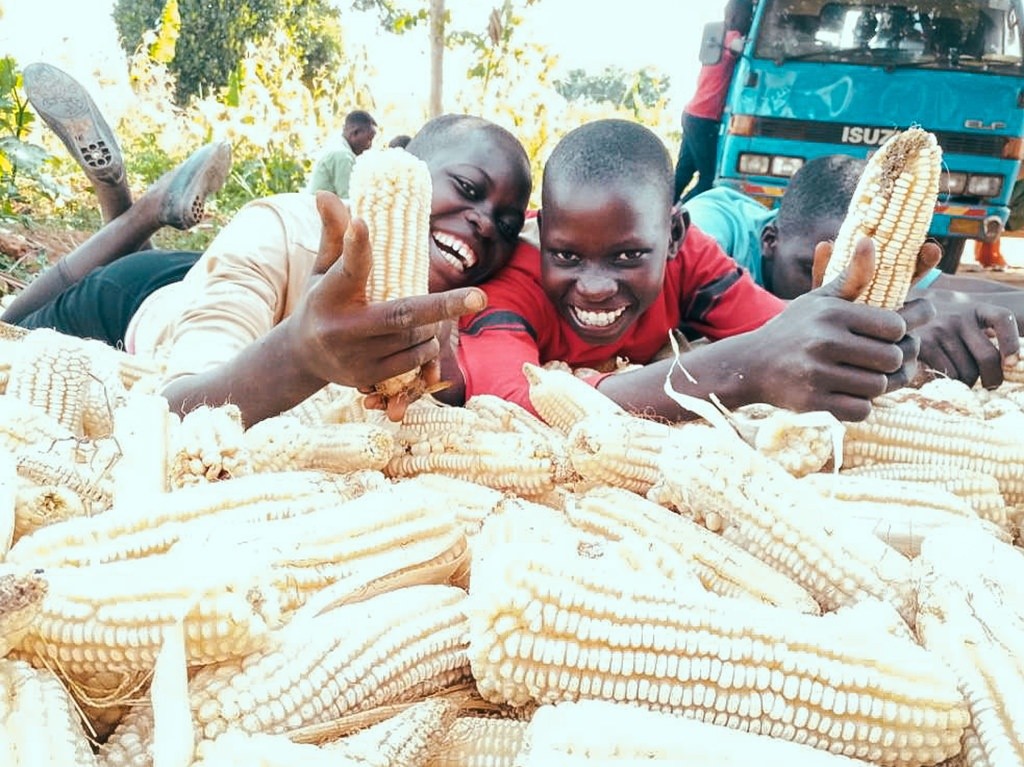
[0,63,535,432]
[454,119,949,428]
[389,134,411,150]
[679,154,1024,394]
[307,110,379,199]
[673,0,755,210]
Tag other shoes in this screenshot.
[22,62,123,186]
[162,139,232,230]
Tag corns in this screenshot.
[819,126,943,310]
[349,147,432,398]
[0,321,1024,767]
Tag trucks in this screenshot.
[700,1,1024,276]
